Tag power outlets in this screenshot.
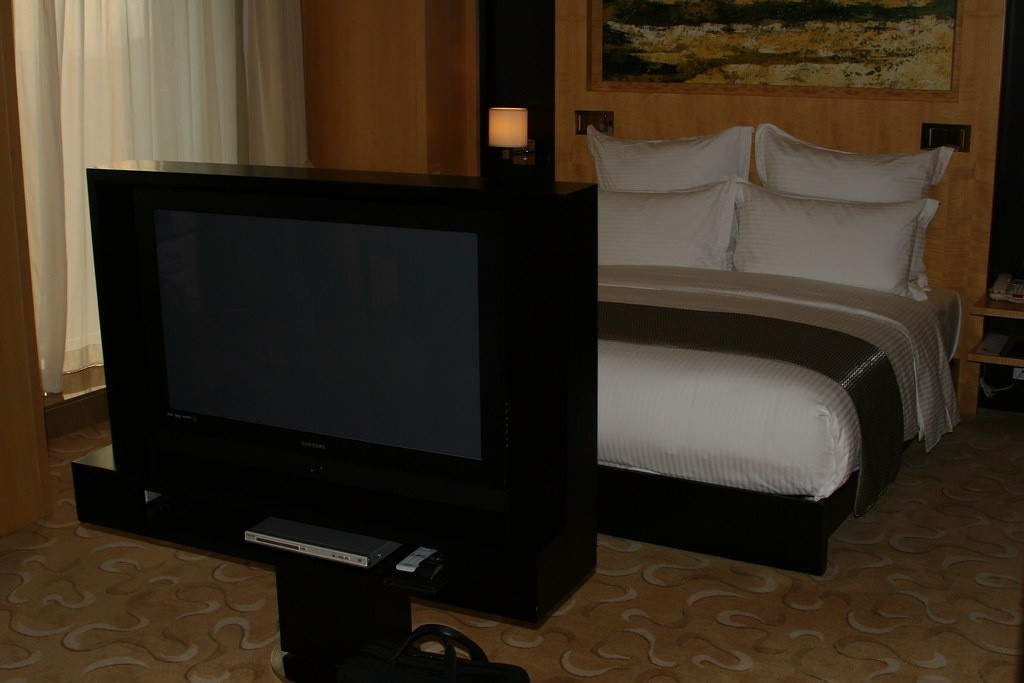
[1013,368,1024,380]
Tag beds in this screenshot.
[596,262,964,575]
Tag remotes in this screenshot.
[396,546,455,581]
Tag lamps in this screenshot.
[487,108,536,165]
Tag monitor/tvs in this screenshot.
[83,158,597,545]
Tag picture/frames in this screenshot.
[591,0,964,102]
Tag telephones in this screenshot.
[987,273,1024,304]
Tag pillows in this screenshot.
[587,124,755,192]
[598,179,741,271]
[731,176,941,302]
[754,123,955,292]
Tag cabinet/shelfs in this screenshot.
[70,443,595,683]
[968,293,1024,367]
